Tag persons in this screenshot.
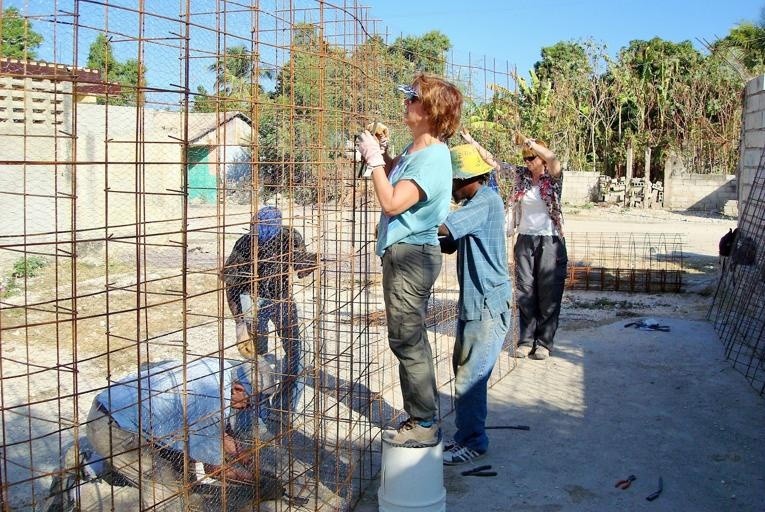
[39,353,278,512]
[217,204,324,427]
[459,123,569,359]
[355,71,465,449]
[374,125,513,468]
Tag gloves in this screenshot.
[358,122,389,169]
[234,321,256,361]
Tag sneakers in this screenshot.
[381,417,442,448]
[515,343,550,359]
[41,445,92,512]
[443,438,487,466]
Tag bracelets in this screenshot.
[523,138,537,149]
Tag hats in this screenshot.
[250,206,282,244]
[445,144,493,182]
[237,366,271,423]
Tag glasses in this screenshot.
[522,154,539,162]
